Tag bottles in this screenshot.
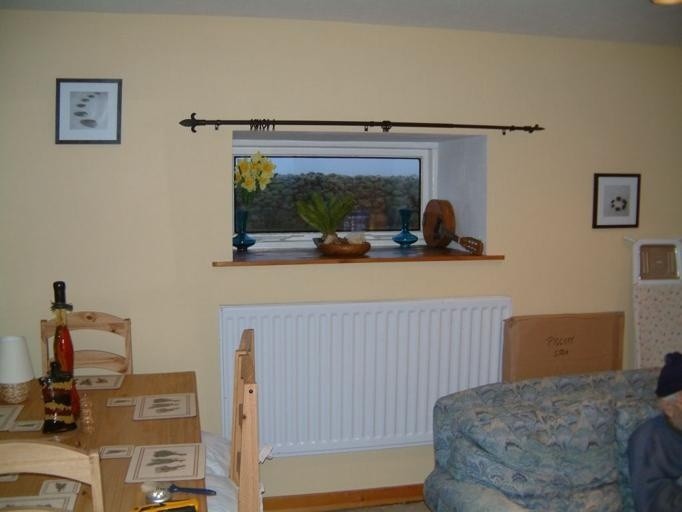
[39,361,78,434]
[47,281,73,422]
[76,392,95,454]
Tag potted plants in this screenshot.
[295,191,371,256]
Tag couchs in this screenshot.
[423,367,682,512]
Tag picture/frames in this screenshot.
[592,172,640,229]
[54,77,123,145]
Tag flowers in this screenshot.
[235,151,276,205]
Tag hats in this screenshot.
[654,351,682,397]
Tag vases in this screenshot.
[233,206,256,253]
[392,210,418,252]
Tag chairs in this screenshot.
[38,307,135,376]
[200,328,258,486]
[201,354,266,512]
[0,440,107,512]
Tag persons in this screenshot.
[628,352,682,512]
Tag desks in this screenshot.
[0,370,208,512]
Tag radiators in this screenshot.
[219,297,512,458]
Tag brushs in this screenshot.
[139,479,218,496]
